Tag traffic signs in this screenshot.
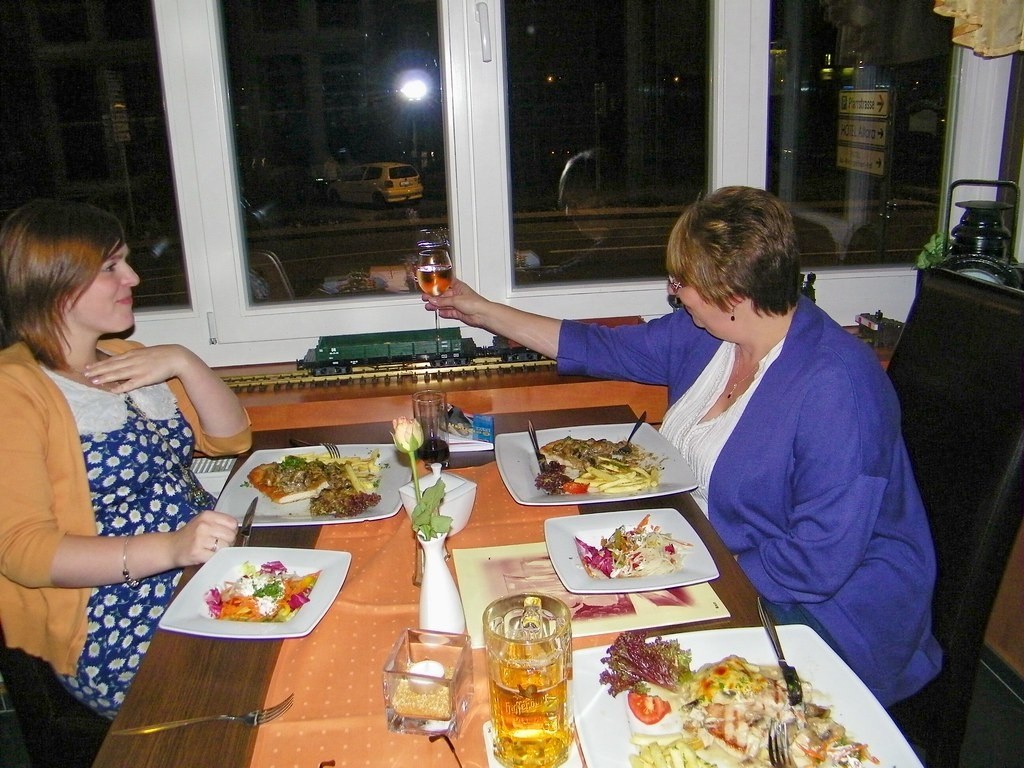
[835,147,886,176]
[838,89,891,117]
[838,119,889,150]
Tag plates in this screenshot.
[214,443,413,526]
[158,547,352,629]
[494,422,697,506]
[573,623,926,768]
[544,508,719,594]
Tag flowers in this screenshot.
[389,416,454,542]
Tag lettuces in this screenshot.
[597,631,694,697]
[534,461,575,491]
[309,486,382,518]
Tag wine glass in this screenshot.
[418,242,453,352]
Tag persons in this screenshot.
[424,187,945,710]
[0,198,253,721]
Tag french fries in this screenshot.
[578,457,662,494]
[294,453,380,494]
[628,732,712,768]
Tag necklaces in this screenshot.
[78,369,207,507]
[727,367,757,399]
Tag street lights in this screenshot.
[402,81,427,168]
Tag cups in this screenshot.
[412,389,450,461]
[380,626,475,739]
[483,592,571,768]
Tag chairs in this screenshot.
[0,623,113,768]
[887,266,1023,768]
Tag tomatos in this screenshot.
[627,692,670,725]
[562,481,591,494]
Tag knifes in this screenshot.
[757,596,803,707]
[240,496,258,547]
[529,420,548,474]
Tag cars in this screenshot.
[318,162,424,212]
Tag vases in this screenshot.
[415,528,466,635]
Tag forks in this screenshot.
[768,718,792,768]
[291,437,340,459]
[613,411,647,455]
[113,692,294,737]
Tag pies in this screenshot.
[248,462,329,502]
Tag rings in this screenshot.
[211,539,218,551]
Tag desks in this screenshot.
[210,364,669,431]
[91,404,760,768]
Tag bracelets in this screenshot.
[122,535,139,587]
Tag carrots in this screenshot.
[220,576,311,622]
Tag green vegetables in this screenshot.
[277,455,307,469]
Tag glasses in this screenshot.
[667,274,685,294]
